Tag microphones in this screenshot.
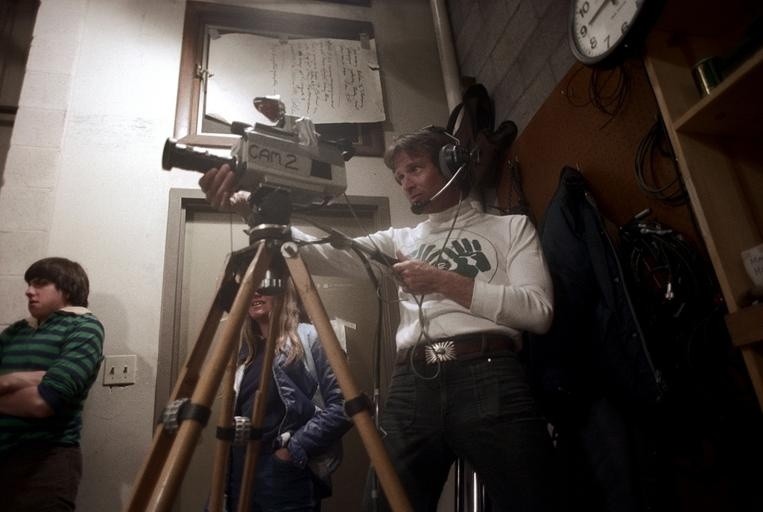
[411,164,465,214]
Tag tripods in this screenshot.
[120,239,414,512]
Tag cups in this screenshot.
[690,53,724,100]
[740,244,763,287]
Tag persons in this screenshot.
[197,124,558,511]
[0,255,106,511]
[219,268,355,511]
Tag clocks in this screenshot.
[567,0,645,67]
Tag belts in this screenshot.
[395,330,522,367]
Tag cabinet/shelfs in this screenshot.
[639,0,763,414]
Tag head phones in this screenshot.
[423,125,471,184]
